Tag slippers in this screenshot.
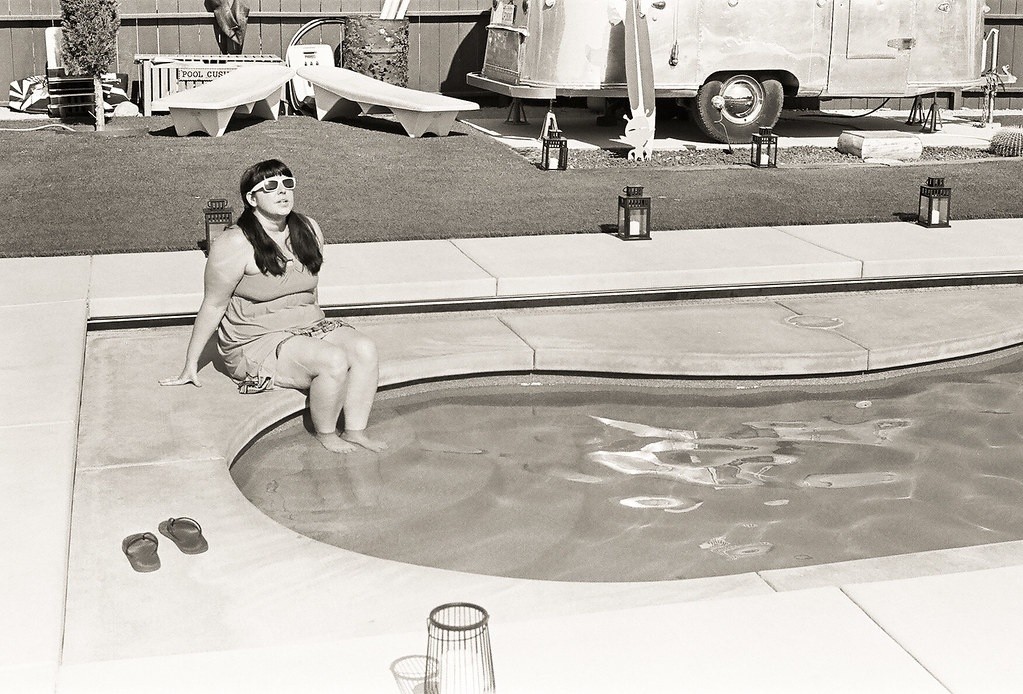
[122,532,160,572]
[159,517,208,555]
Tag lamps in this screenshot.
[616,184,652,241]
[917,177,952,228]
[749,125,778,168]
[540,129,567,170]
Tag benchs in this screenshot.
[150,62,296,138]
[295,64,481,139]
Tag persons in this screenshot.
[159,159,387,454]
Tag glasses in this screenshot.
[250,176,296,194]
